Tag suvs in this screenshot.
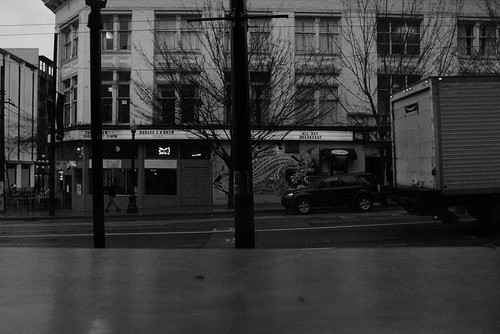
[281,171,380,214]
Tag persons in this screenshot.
[105,183,122,212]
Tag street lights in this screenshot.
[35,152,49,192]
[128,116,136,214]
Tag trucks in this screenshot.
[390,72,499,213]
